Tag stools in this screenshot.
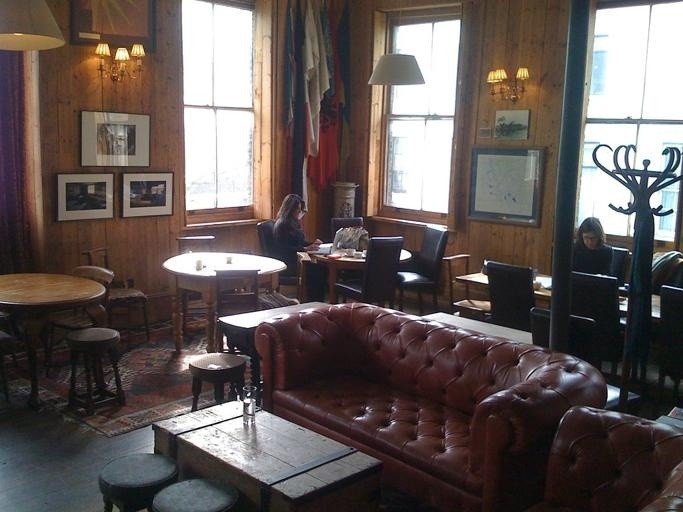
[187,350,248,410]
[61,325,128,416]
[148,475,242,512]
[95,453,182,511]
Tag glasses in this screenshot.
[294,209,301,212]
[583,236,596,239]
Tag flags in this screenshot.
[281,0,354,228]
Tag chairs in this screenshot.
[524,404,682,511]
[0,245,151,410]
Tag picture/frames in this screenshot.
[54,171,115,222]
[466,146,547,229]
[121,170,174,219]
[79,109,152,169]
[69,0,158,54]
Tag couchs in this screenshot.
[251,301,610,511]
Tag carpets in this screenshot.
[0,308,264,440]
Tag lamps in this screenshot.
[0,0,67,53]
[486,66,530,105]
[366,0,426,87]
[95,42,145,84]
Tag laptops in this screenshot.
[308,227,343,255]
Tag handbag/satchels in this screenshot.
[333,227,368,250]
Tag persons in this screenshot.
[569,217,614,278]
[271,193,327,303]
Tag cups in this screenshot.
[344,248,363,259]
[242,386,256,426]
